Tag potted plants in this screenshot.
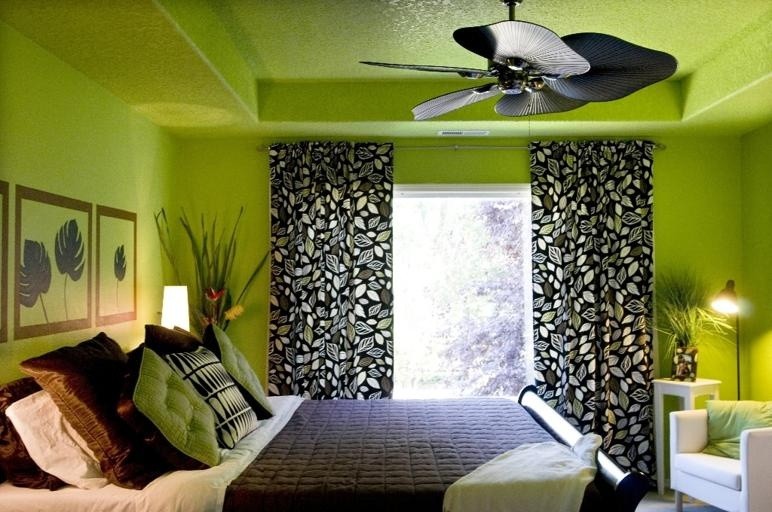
[645,268,735,383]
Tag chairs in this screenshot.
[664,402,771,512]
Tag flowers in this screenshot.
[153,198,275,344]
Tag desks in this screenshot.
[646,374,724,504]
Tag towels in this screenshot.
[437,430,604,511]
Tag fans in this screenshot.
[357,0,684,125]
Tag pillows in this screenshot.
[210,323,275,416]
[165,346,258,450]
[4,389,111,489]
[698,399,771,460]
[133,347,221,467]
[16,331,210,489]
[145,325,202,355]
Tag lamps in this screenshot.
[160,281,191,333]
[708,276,745,402]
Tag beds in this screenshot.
[3,340,653,511]
